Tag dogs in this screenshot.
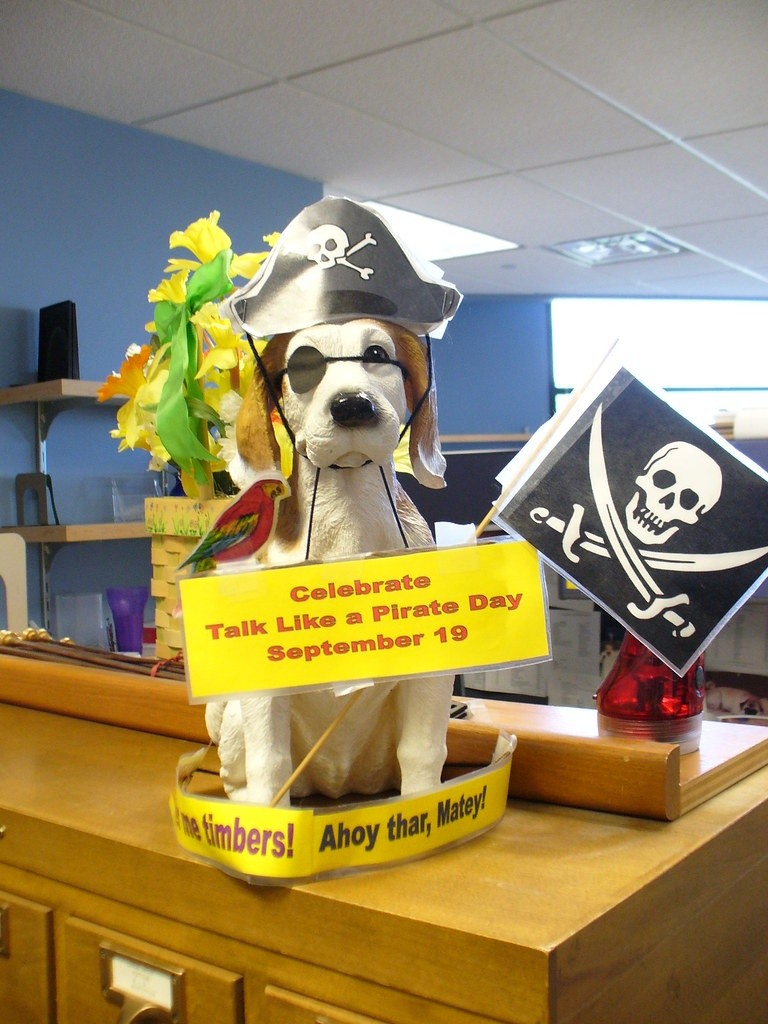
[203,316,458,809]
[705,681,768,722]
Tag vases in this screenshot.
[144,496,239,666]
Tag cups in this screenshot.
[106,587,149,656]
[56,592,103,654]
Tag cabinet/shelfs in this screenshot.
[0,378,150,542]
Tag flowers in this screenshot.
[97,210,415,500]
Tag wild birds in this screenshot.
[177,478,288,574]
